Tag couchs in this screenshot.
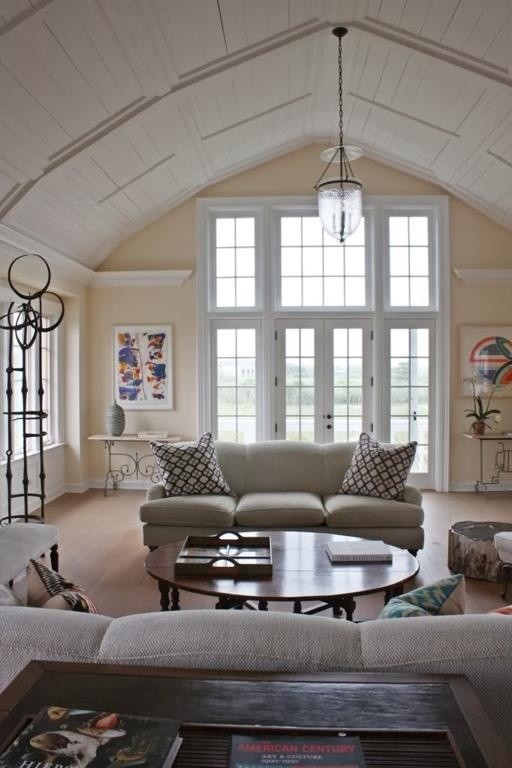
[139,438,424,558]
[0,578,512,741]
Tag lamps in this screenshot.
[315,26,364,243]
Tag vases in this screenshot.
[105,401,125,436]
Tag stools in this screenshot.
[0,522,59,586]
[449,520,512,582]
[494,531,512,597]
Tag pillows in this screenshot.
[149,433,235,497]
[26,559,99,616]
[376,573,466,621]
[339,433,378,480]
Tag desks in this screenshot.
[87,432,182,497]
[0,655,512,768]
[461,431,512,499]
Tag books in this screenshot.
[502,431,511,436]
[230,733,365,768]
[326,539,394,562]
[138,432,168,440]
[1,705,186,768]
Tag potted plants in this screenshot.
[458,374,504,434]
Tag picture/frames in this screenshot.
[458,323,512,398]
[112,323,174,411]
